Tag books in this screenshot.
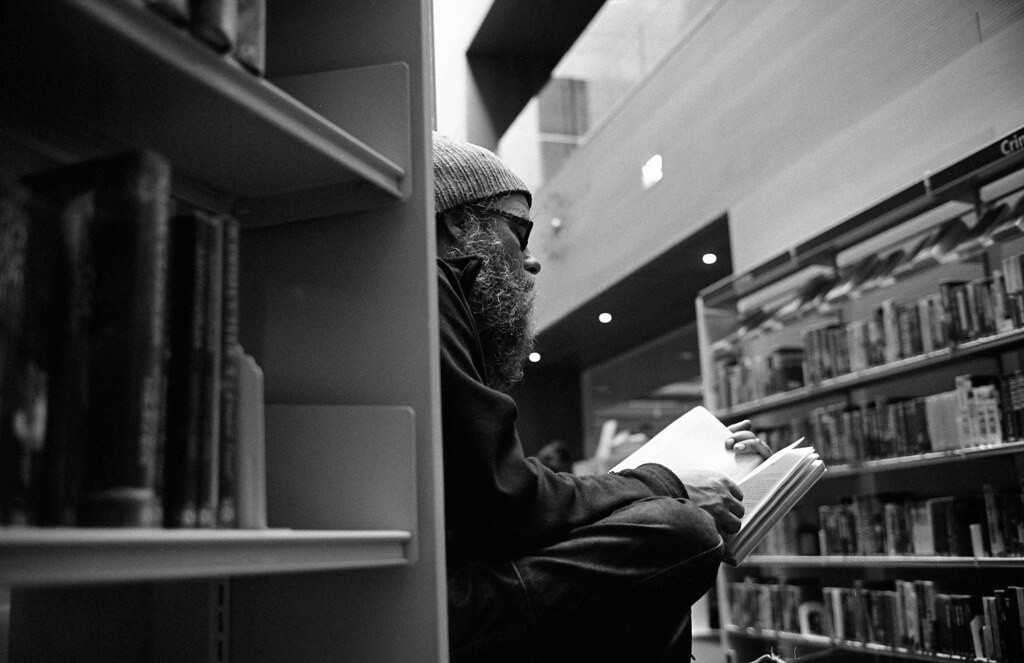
[607,405,828,568]
[712,251,1024,661]
[151,0,267,77]
[2,147,267,529]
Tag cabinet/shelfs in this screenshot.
[0,0,451,663]
[694,124,1024,663]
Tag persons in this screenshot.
[432,131,773,663]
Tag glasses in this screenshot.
[469,201,533,251]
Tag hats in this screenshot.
[432,130,533,214]
